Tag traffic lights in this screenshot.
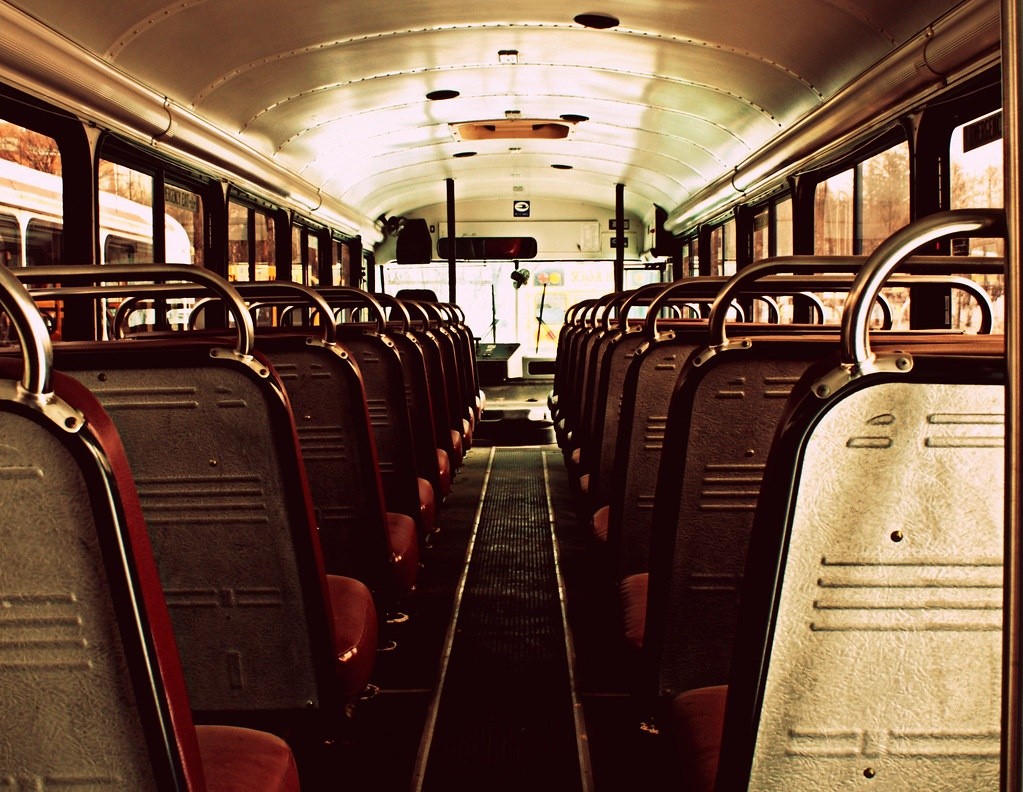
[534,271,563,286]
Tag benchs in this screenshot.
[0,264,487,792]
[549,207,1007,791]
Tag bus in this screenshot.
[0,157,194,333]
[224,217,322,326]
